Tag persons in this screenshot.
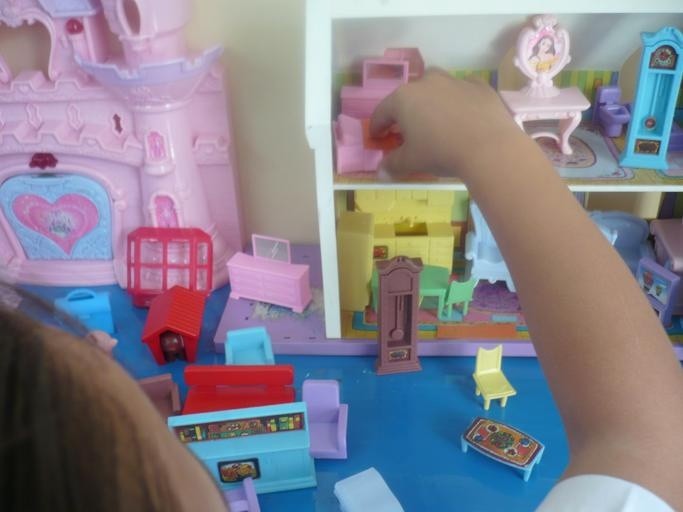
[1,74,682,511]
